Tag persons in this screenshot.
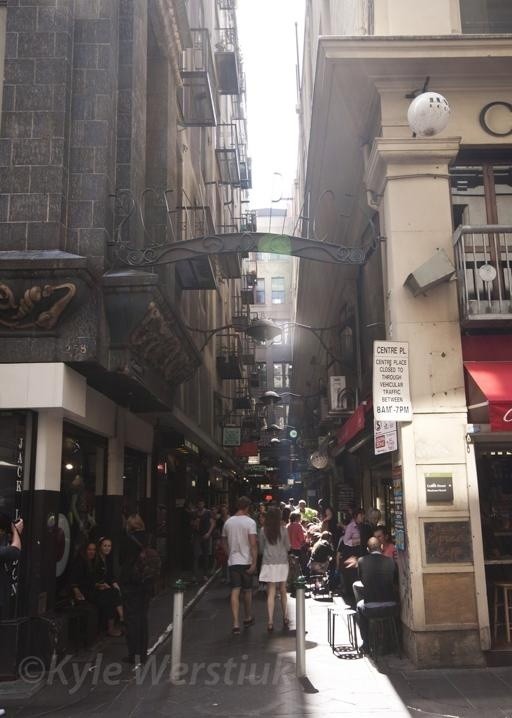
[1,518,25,617]
[60,494,400,671]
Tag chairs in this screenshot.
[0,577,124,681]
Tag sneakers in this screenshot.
[232,618,290,635]
[122,654,146,663]
[109,619,126,637]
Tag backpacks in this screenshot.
[133,534,162,585]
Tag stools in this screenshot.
[491,581,512,642]
[310,574,403,658]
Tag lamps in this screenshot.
[309,432,338,469]
[405,76,453,137]
[186,318,283,448]
